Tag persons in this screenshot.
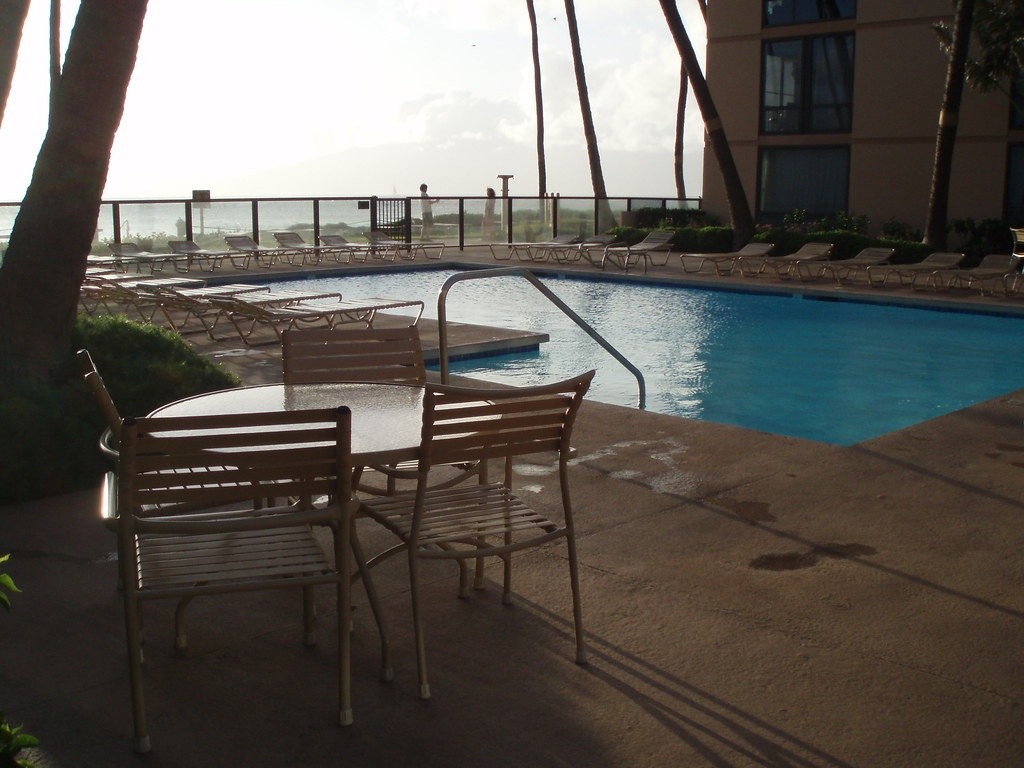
[482,188,496,242]
[419,184,439,243]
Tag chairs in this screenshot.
[78,227,1024,755]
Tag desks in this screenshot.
[144,377,503,651]
